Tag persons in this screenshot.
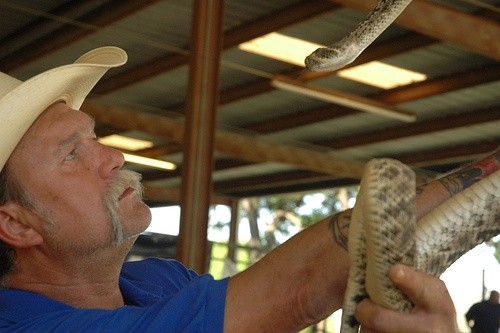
[0,46,500,333]
[464,291,500,333]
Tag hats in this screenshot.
[0,46,128,175]
